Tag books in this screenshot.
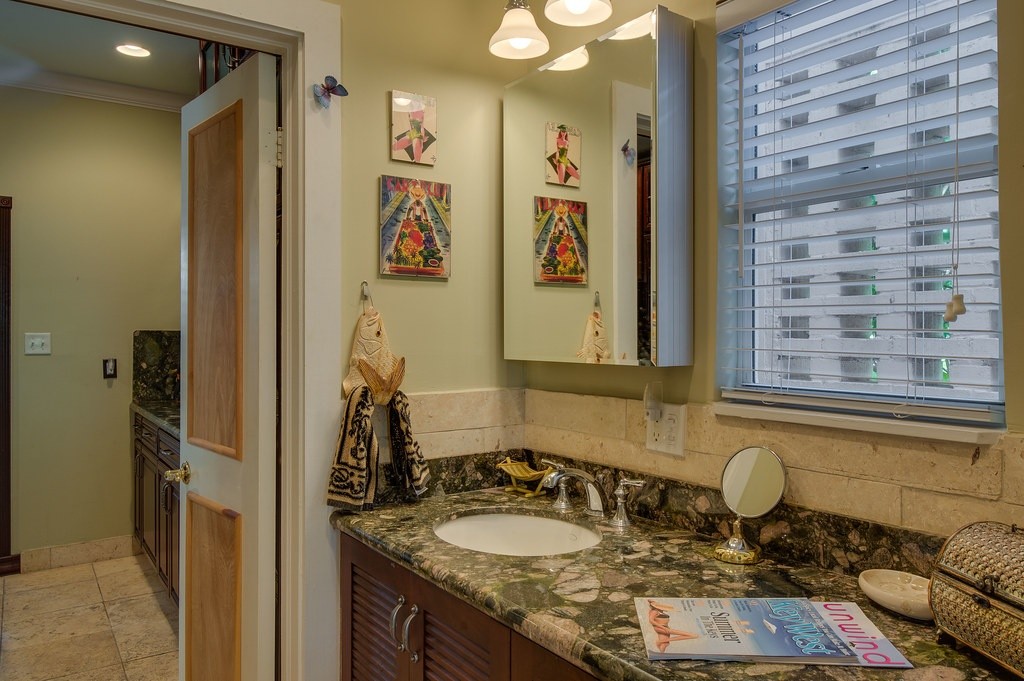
[635,597,916,668]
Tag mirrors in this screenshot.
[503,8,658,365]
[714,445,785,561]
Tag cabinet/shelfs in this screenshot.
[339,528,599,681]
[504,3,693,368]
[132,412,181,611]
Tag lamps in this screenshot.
[547,13,655,72]
[489,1,550,60]
[542,1,613,26]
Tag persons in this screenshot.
[391,101,430,162]
[553,124,581,185]
[647,599,698,653]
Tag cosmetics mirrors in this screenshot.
[717,444,787,565]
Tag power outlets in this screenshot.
[645,402,687,457]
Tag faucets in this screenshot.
[541,468,613,517]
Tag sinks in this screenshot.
[431,507,603,557]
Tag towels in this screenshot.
[326,383,433,516]
